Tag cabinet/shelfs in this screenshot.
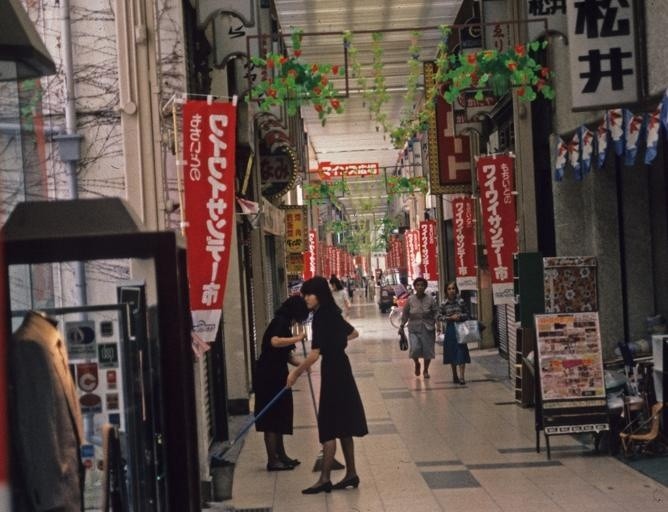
[512,252,555,410]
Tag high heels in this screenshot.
[302,481,330,494]
[332,476,359,488]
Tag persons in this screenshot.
[327,269,377,324]
[252,294,310,472]
[5,307,85,511]
[397,277,442,379]
[300,276,369,494]
[435,280,471,385]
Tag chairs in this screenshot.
[618,401,668,459]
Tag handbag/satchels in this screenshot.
[399,332,408,351]
[454,317,486,344]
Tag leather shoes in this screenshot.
[267,458,300,470]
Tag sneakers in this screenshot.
[415,363,430,378]
[453,377,465,384]
